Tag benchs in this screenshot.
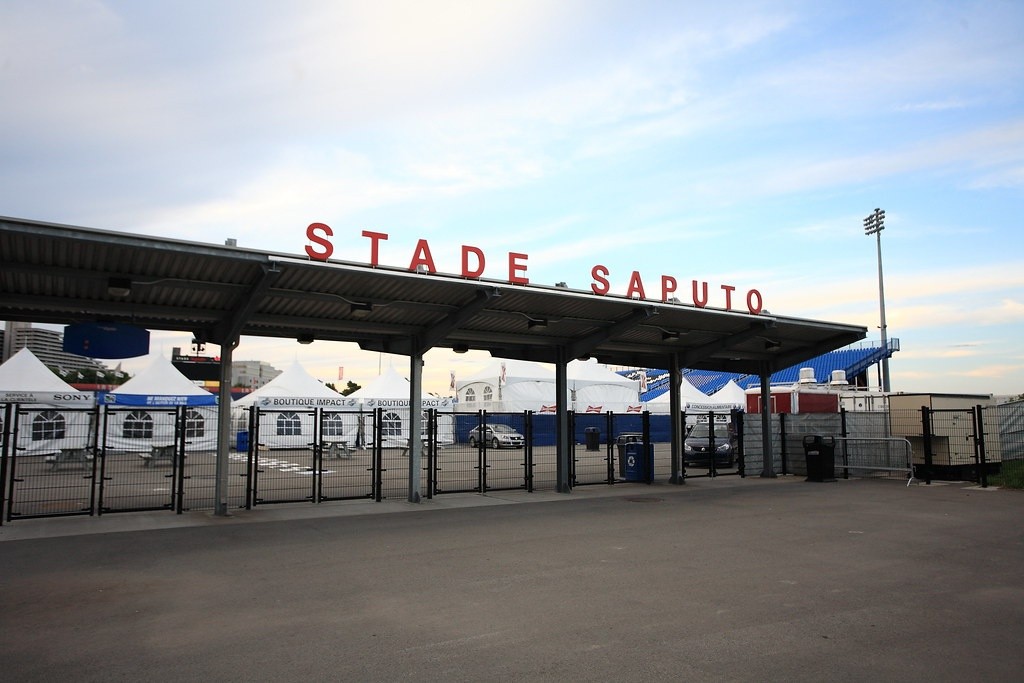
[139,453,155,468]
[83,454,94,470]
[397,445,425,457]
[309,447,332,461]
[171,453,188,467]
[336,447,357,460]
[45,456,58,471]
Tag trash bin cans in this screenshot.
[802,435,838,483]
[617,434,643,477]
[584,426,601,450]
[625,442,655,483]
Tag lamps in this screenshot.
[662,331,680,343]
[350,301,372,318]
[765,341,784,353]
[106,277,132,297]
[527,318,548,332]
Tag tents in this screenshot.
[0,347,747,456]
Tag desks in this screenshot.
[405,447,433,456]
[150,445,175,466]
[57,448,85,469]
[323,440,351,460]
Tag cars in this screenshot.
[468,421,525,449]
[682,421,739,468]
